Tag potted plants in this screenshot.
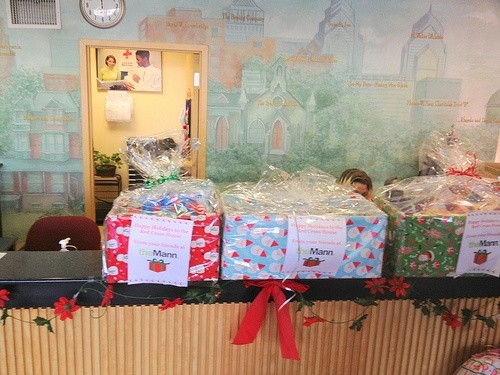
[93,148,123,177]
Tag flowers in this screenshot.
[0,279,500,336]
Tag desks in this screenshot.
[0,249,500,375]
[94,174,122,221]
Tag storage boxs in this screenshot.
[376,188,500,278]
[221,186,389,279]
[105,188,219,283]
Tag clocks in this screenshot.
[79,0,127,29]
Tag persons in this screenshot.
[337,168,373,202]
[98,55,121,81]
[123,50,161,91]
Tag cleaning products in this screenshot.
[59,237,71,251]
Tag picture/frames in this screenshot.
[96,49,164,93]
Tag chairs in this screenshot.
[19,215,103,250]
